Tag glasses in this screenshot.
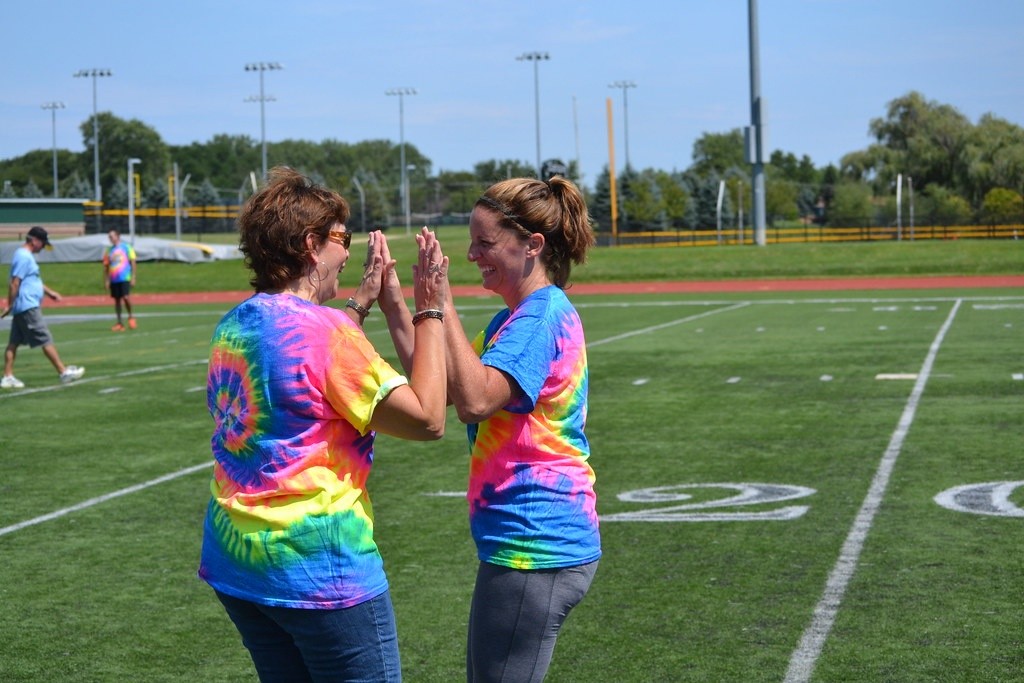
[328,229,351,249]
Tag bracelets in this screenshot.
[413,308,444,318]
[345,295,370,318]
[411,311,444,326]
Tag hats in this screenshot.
[28,227,51,247]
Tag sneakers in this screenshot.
[0,376,25,388]
[128,318,136,328]
[111,323,125,331]
[58,364,85,384]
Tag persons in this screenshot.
[364,174,604,683]
[101,230,138,332]
[198,167,452,683]
[0,226,86,388]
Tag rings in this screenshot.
[428,264,439,275]
[363,262,368,269]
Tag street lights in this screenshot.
[73,67,113,235]
[40,100,67,200]
[513,48,552,183]
[385,86,419,218]
[607,78,639,176]
[243,59,285,190]
